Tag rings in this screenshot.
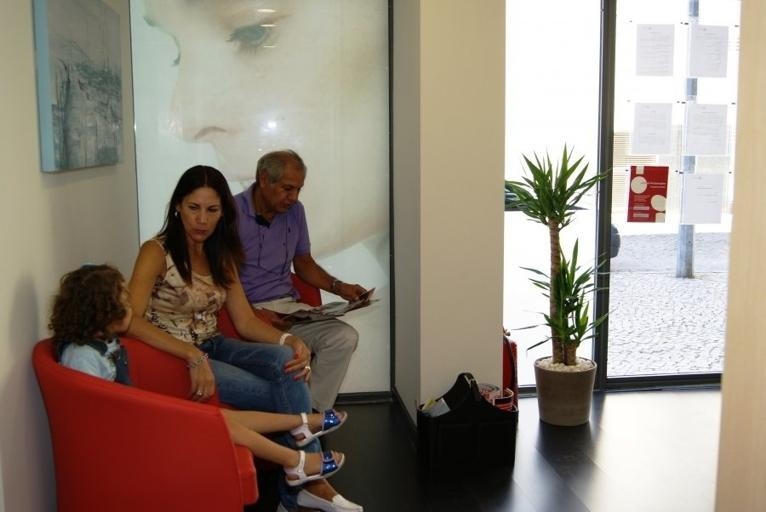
[197,391,203,397]
[305,365,311,371]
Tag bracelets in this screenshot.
[184,353,210,369]
[331,278,342,294]
[279,333,292,346]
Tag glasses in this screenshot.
[257,248,288,276]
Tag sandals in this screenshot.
[283,449,345,486]
[290,408,348,448]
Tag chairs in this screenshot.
[32,333,260,511]
[214,272,322,341]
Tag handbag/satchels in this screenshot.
[416,372,519,497]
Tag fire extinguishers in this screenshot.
[502,325,517,410]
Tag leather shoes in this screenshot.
[296,485,364,512]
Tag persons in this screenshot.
[48,264,349,488]
[238,149,370,412]
[124,164,364,512]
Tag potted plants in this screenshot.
[505,144,618,427]
